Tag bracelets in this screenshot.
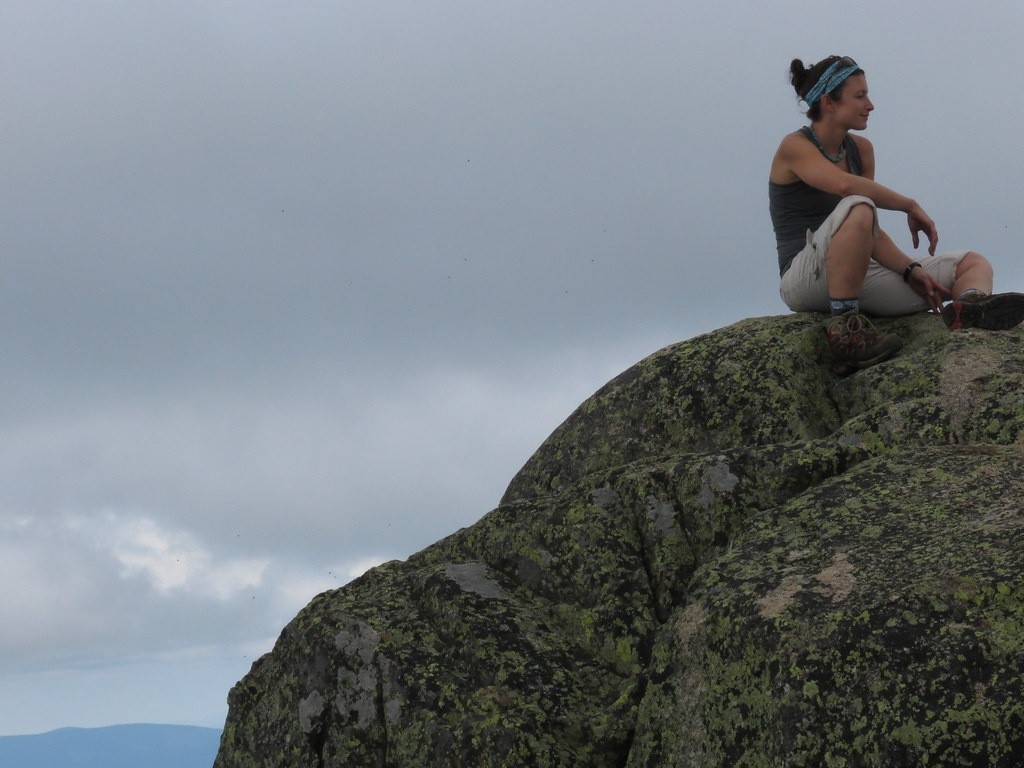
[903,262,922,283]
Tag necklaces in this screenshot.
[810,123,844,162]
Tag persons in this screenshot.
[768,55,1024,377]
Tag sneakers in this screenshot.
[942,292,1024,331]
[826,308,903,377]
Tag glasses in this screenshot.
[823,56,859,95]
